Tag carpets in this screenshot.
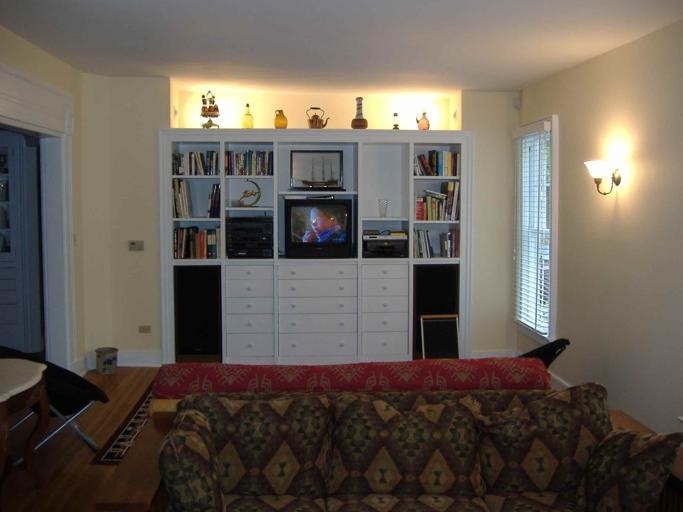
[89,367,161,465]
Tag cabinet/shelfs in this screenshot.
[159,129,474,365]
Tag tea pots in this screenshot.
[305,106,331,129]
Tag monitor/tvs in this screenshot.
[284,198,353,258]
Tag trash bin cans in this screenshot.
[95,346,119,376]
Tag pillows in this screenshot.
[575,428,683,511]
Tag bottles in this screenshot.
[415,111,431,130]
[350,96,369,129]
[242,102,254,129]
[273,109,289,128]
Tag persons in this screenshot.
[302,206,344,241]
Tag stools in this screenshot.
[0,358,52,491]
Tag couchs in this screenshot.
[148,383,683,512]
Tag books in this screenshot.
[172,148,273,260]
[412,149,460,258]
